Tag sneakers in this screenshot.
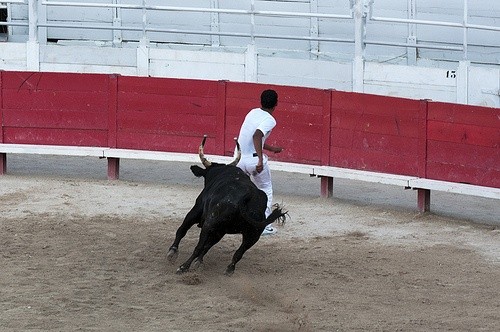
[261,227,277,235]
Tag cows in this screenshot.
[166,134,291,275]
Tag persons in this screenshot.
[234,89,282,235]
